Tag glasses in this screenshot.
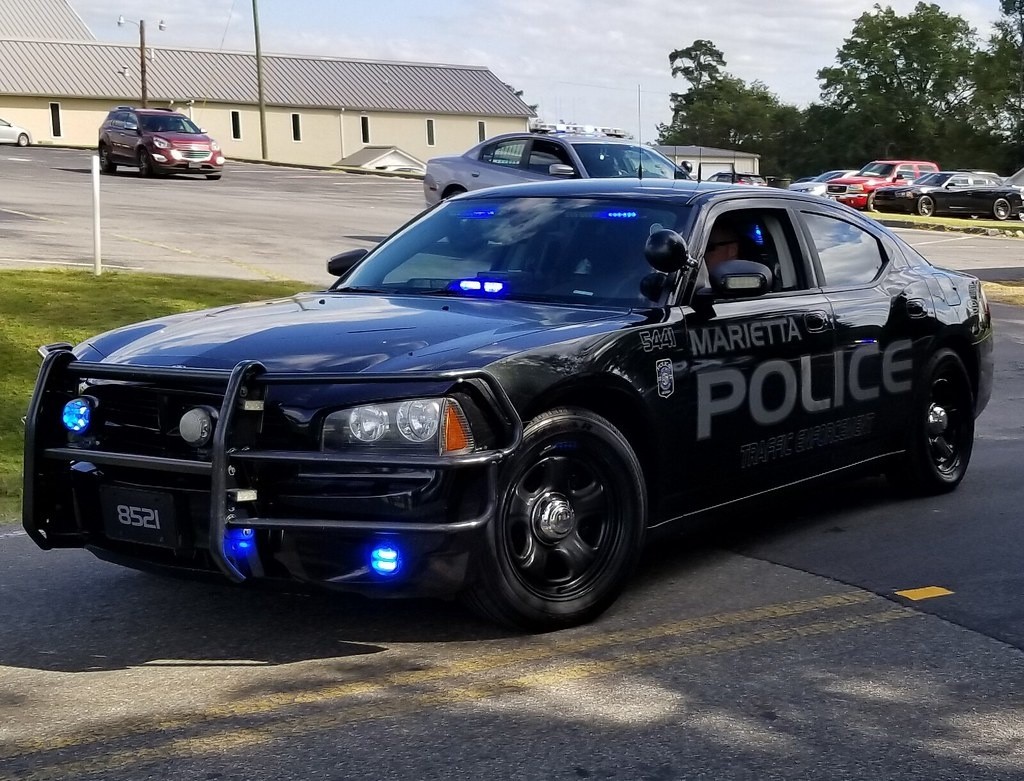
[706,240,736,251]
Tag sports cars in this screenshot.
[19,169,996,634]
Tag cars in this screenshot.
[0,118,32,147]
[423,120,701,253]
[706,159,1024,222]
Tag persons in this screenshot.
[704,220,744,273]
[173,120,184,131]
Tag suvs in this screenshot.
[97,105,226,180]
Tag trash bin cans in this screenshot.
[765,176,791,190]
